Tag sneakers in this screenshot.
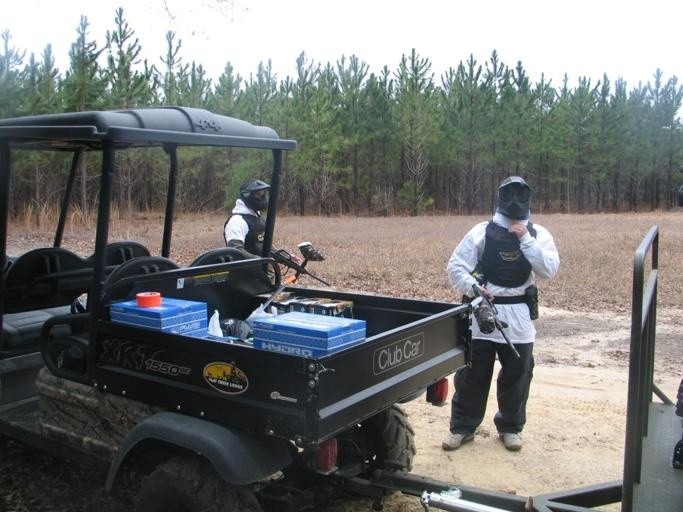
[499,432,522,451]
[442,432,474,450]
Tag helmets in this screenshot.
[240,178,271,211]
[497,176,535,220]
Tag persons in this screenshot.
[222,177,302,281]
[438,174,561,453]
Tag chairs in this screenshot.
[1,241,246,388]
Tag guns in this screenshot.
[269,243,329,286]
[471,285,520,359]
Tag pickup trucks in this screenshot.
[1,103,474,512]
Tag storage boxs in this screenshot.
[109,297,366,361]
